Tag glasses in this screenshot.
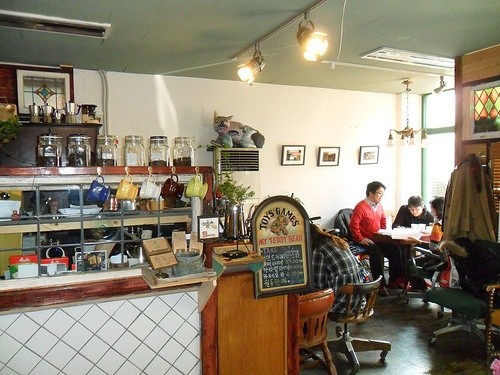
[375,191,384,196]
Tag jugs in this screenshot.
[29,101,81,123]
[172,249,206,276]
[216,200,254,239]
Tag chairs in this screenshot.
[426,286,487,345]
[295,288,338,375]
[327,274,392,375]
[402,245,440,300]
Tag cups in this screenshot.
[185,173,209,201]
[139,174,162,201]
[161,174,184,199]
[411,223,433,233]
[103,194,120,212]
[123,199,141,213]
[116,174,139,203]
[81,104,98,113]
[85,175,111,206]
[146,196,164,211]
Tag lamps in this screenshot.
[386,80,431,150]
[237,43,265,84]
[297,11,329,61]
[432,75,448,94]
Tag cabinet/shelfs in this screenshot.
[1,165,213,292]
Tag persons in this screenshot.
[349,181,447,295]
[300,222,368,364]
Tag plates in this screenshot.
[56,207,103,217]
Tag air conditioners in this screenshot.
[212,147,261,232]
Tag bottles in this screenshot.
[173,137,193,166]
[95,135,119,166]
[123,135,146,166]
[67,134,92,167]
[37,132,62,167]
[149,136,170,166]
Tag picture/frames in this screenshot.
[318,146,340,166]
[282,145,306,166]
[359,145,380,165]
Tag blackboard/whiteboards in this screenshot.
[260,244,305,289]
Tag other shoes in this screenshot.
[389,276,405,289]
[377,286,389,296]
